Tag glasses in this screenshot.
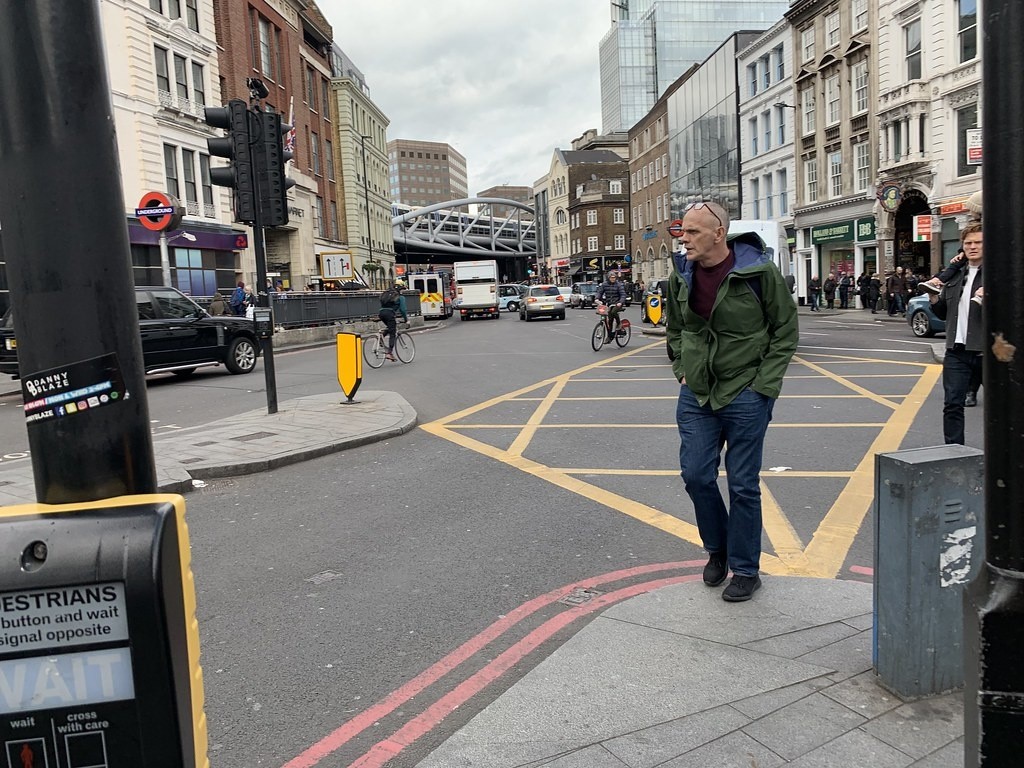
[685,201,723,227]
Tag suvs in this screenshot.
[571,280,602,309]
[0,285,263,391]
[642,278,674,325]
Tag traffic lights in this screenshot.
[260,112,301,229]
[200,96,258,223]
[253,309,274,338]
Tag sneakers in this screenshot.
[703,547,728,587]
[722,575,761,601]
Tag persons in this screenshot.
[378,279,410,361]
[666,202,800,601]
[267,278,284,292]
[917,188,983,446]
[858,266,926,316]
[809,271,851,312]
[209,282,256,316]
[634,280,645,291]
[594,272,627,344]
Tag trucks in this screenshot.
[408,270,455,320]
[453,259,502,320]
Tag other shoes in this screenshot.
[892,313,900,317]
[970,295,983,307]
[810,310,815,312]
[816,309,821,313]
[965,392,977,407]
[603,336,611,345]
[616,321,623,331]
[378,336,384,348]
[872,310,878,314]
[918,281,941,295]
[386,353,397,361]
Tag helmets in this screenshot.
[396,280,407,287]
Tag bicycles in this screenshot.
[363,320,417,369]
[592,299,632,352]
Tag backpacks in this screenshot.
[380,289,400,309]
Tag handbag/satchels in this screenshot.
[222,302,230,315]
[825,284,831,292]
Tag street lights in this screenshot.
[360,133,381,286]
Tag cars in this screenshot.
[555,286,576,306]
[908,290,956,337]
[499,284,529,311]
[520,284,567,321]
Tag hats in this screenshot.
[608,272,617,278]
[967,190,984,216]
